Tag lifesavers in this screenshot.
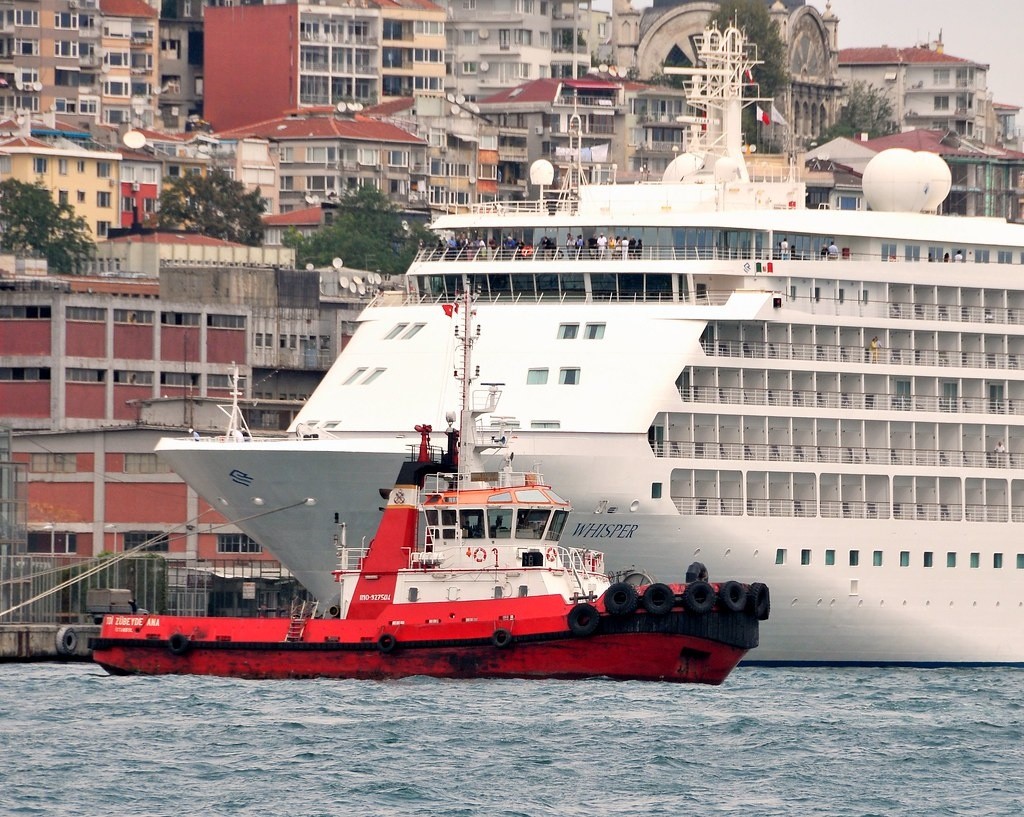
[546,547,558,562]
[719,580,747,613]
[603,581,640,617]
[566,603,601,636]
[640,582,676,616]
[53,625,79,655]
[681,580,717,616]
[491,628,512,648]
[473,548,488,562]
[167,631,190,656]
[376,633,397,653]
[748,582,771,621]
[521,245,534,256]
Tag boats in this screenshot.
[87,280,771,686]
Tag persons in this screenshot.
[501,235,557,260]
[230,427,250,442]
[869,336,882,364]
[781,238,789,260]
[828,241,838,260]
[436,235,497,261]
[189,428,199,441]
[821,244,829,260]
[565,233,642,260]
[954,250,963,263]
[414,239,426,262]
[994,441,1005,468]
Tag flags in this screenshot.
[442,304,454,318]
[757,106,769,125]
[771,104,789,126]
[745,67,754,83]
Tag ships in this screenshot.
[151,9,1024,669]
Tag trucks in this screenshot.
[86,588,150,625]
